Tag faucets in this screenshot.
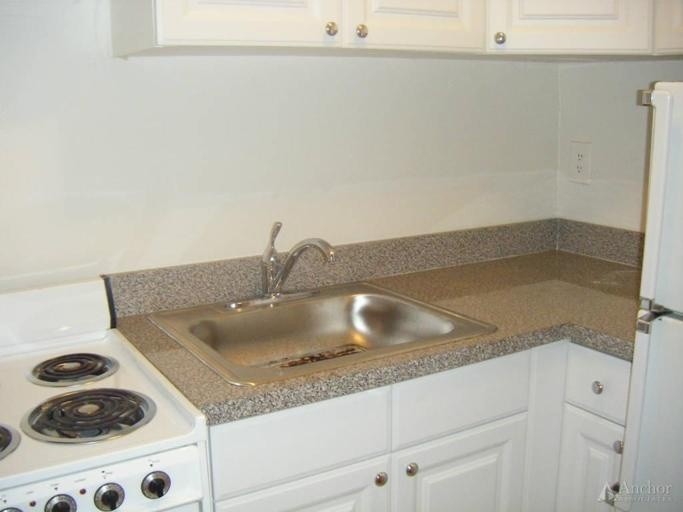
[260,221,339,294]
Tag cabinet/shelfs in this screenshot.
[554,343,633,512]
[209,348,531,512]
[652,1,683,58]
[154,1,486,54]
[486,0,652,56]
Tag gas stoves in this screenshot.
[0,329,211,507]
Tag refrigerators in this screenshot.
[614,81,683,510]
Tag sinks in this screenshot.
[147,280,498,388]
